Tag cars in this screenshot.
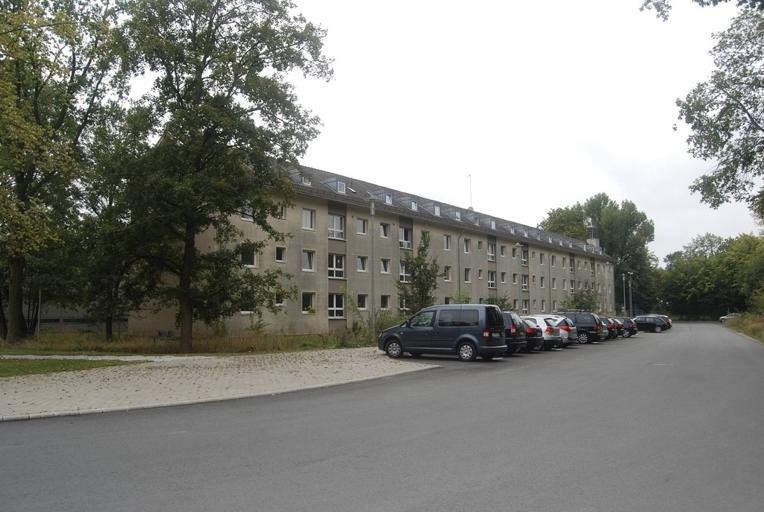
[502,308,673,363]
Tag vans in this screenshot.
[377,302,509,363]
